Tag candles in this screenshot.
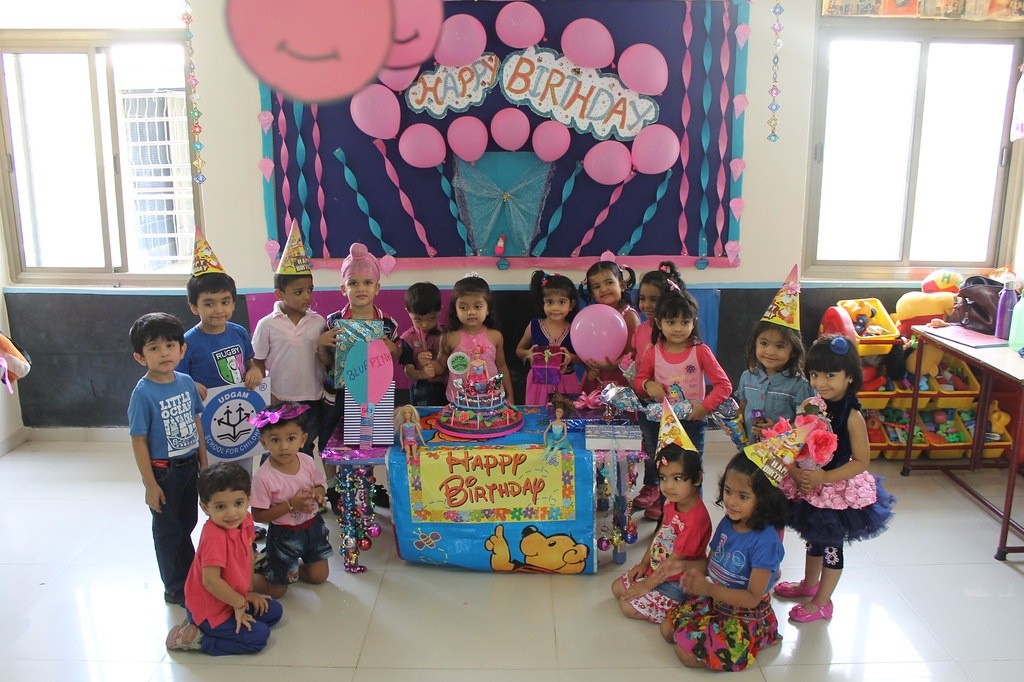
[451,390,454,403]
[477,393,480,407]
[477,414,479,429]
[491,392,494,407]
[507,411,509,425]
[450,410,454,426]
[501,392,502,403]
[464,395,469,407]
[458,393,459,404]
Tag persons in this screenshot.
[317,255,399,517]
[580,261,641,413]
[127,312,208,608]
[611,440,713,624]
[251,274,327,460]
[174,272,266,539]
[633,288,733,537]
[775,336,871,623]
[392,403,428,461]
[399,282,449,406]
[249,401,335,599]
[516,274,580,406]
[660,451,784,672]
[417,277,514,406]
[630,270,679,521]
[541,402,569,462]
[721,321,815,581]
[166,462,283,657]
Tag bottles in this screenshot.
[995,279,1017,339]
[1010,289,1024,352]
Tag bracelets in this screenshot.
[232,598,248,610]
[286,498,295,515]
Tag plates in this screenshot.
[435,412,524,437]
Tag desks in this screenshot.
[901,324,1024,561]
[321,404,650,573]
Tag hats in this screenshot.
[759,264,800,333]
[743,421,816,487]
[656,394,699,455]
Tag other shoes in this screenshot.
[164,589,187,608]
[374,485,390,508]
[774,580,833,623]
[327,487,341,514]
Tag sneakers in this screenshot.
[633,485,663,521]
[166,618,202,652]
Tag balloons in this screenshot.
[350,0,679,185]
[570,303,627,367]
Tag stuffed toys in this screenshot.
[895,269,966,376]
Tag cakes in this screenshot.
[437,380,522,434]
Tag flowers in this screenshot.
[808,395,827,412]
[763,413,838,471]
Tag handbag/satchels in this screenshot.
[954,276,1021,334]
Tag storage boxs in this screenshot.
[547,392,630,432]
[344,381,395,444]
[531,345,563,384]
[334,319,384,389]
[836,298,1011,461]
[585,425,643,451]
